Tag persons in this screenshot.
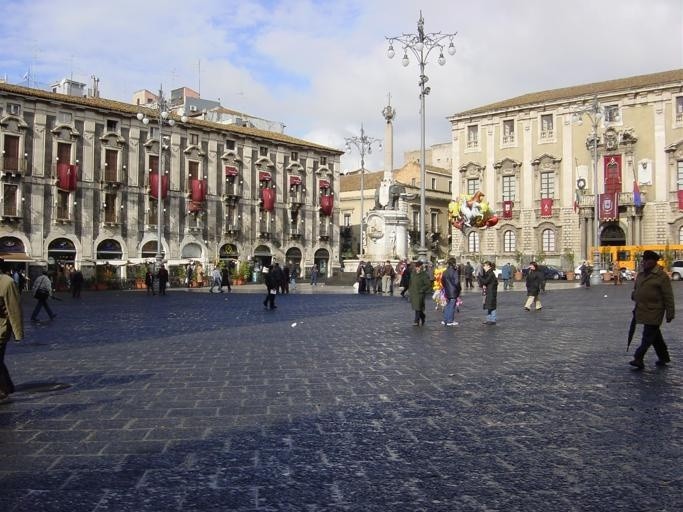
[184,262,231,294]
[441,258,462,326]
[144,268,155,296]
[156,265,169,294]
[356,261,622,298]
[262,259,297,309]
[477,261,498,325]
[6,268,83,322]
[310,264,319,285]
[409,261,431,326]
[628,250,675,367]
[524,262,546,311]
[0,257,24,401]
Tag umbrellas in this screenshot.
[627,305,637,354]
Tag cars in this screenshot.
[434,260,683,281]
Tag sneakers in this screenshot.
[656,358,670,365]
[629,360,644,368]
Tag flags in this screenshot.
[632,180,641,208]
[573,189,580,213]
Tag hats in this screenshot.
[641,251,660,260]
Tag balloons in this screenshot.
[446,192,499,236]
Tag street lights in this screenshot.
[383,11,457,272]
[136,83,189,289]
[571,92,620,286]
[342,119,383,260]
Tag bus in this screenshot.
[588,245,683,272]
